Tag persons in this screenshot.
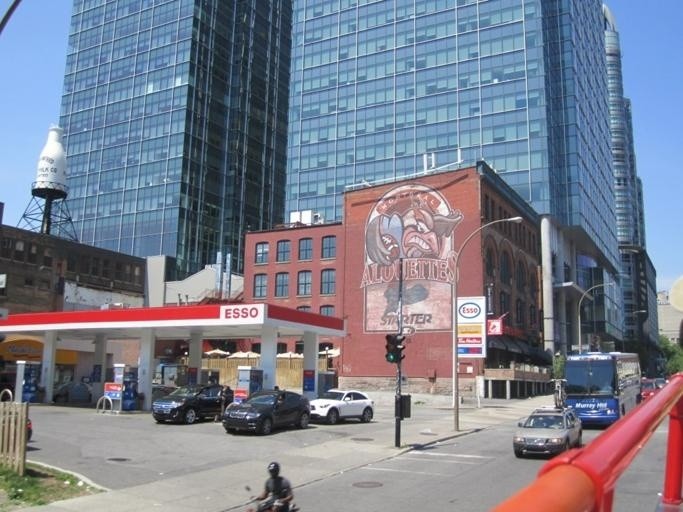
[274,385,283,400]
[214,386,230,423]
[249,462,294,512]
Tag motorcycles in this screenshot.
[244,485,300,512]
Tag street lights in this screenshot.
[622,310,646,353]
[578,282,616,355]
[452,216,523,432]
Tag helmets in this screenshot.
[268,462,279,471]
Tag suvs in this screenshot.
[152,384,233,425]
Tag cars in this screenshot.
[53,383,92,405]
[0,409,33,453]
[308,389,376,425]
[640,376,669,399]
[513,406,583,459]
[222,390,311,436]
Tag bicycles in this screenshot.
[547,379,567,408]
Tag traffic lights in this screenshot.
[385,333,406,364]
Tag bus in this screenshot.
[563,351,642,429]
